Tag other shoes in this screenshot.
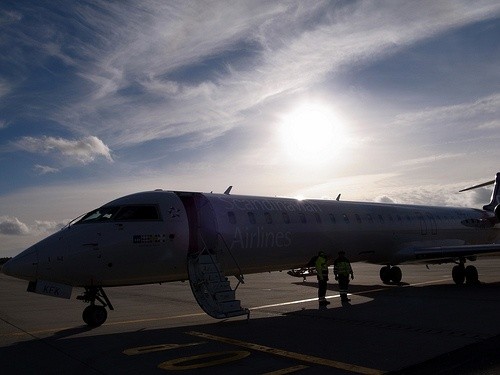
[319,301,330,304]
[341,297,351,301]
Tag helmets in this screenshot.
[337,246,346,252]
[319,248,328,255]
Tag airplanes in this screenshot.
[0,168,500,327]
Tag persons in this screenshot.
[332,250,354,303]
[315,250,331,307]
